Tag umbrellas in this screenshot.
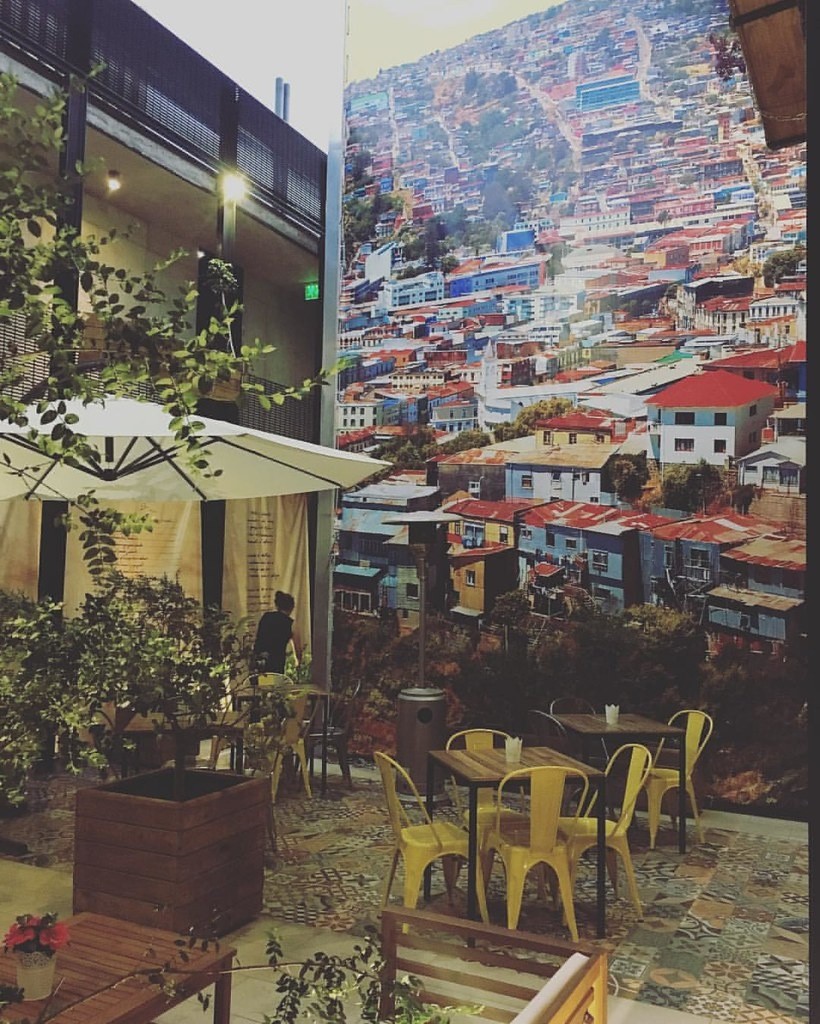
[0,391,393,502]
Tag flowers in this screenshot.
[3,912,72,959]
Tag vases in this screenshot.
[18,953,58,1002]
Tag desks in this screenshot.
[231,684,327,795]
[550,712,687,854]
[424,746,606,948]
[0,911,236,1024]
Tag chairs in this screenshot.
[207,671,714,944]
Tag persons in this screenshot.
[248,591,299,724]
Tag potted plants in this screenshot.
[0,569,314,936]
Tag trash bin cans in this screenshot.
[395,687,453,809]
